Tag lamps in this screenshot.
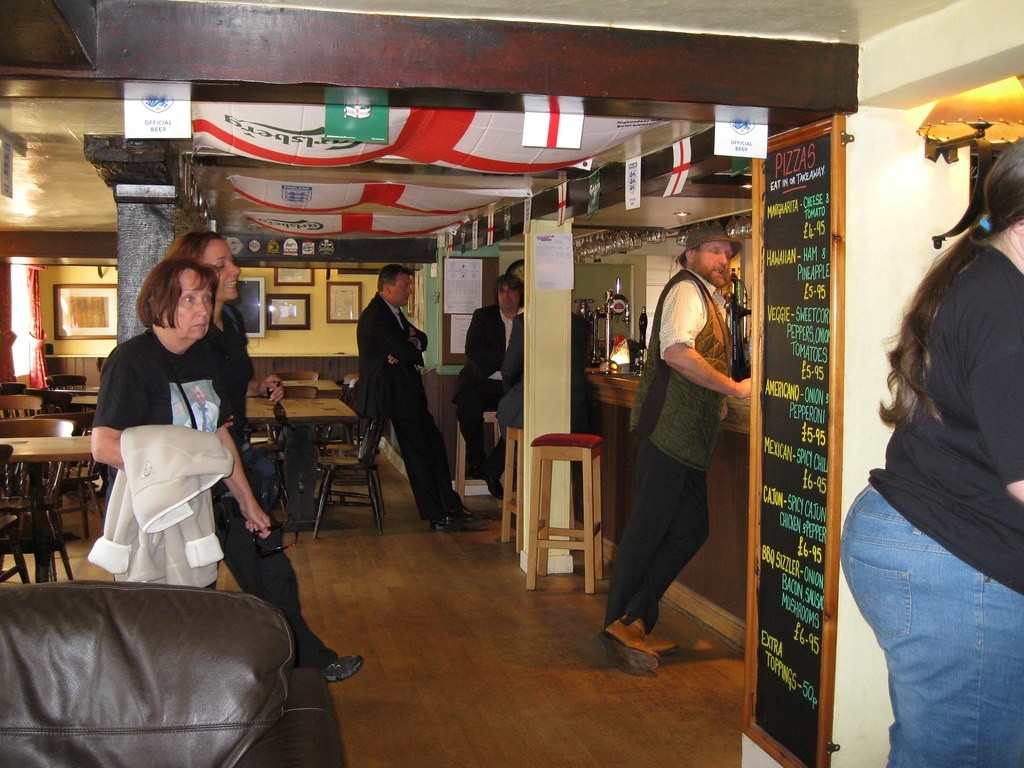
[915,77,1024,165]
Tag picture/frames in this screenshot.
[231,276,265,338]
[53,283,119,340]
[266,292,311,330]
[273,267,315,286]
[325,281,362,323]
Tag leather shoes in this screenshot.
[468,465,483,479]
[484,472,503,501]
[430,505,498,530]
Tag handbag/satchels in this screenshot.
[211,446,289,532]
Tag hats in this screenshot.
[678,225,742,267]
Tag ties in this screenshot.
[199,407,208,432]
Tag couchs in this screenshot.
[0,578,353,768]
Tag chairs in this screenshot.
[0,370,391,585]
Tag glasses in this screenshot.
[267,381,288,425]
[251,514,299,556]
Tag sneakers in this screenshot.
[322,649,362,682]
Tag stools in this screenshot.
[455,410,500,503]
[525,433,605,592]
[501,426,524,553]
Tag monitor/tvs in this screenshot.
[226,277,265,338]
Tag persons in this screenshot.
[453,275,594,499]
[355,264,493,532]
[90,260,272,592]
[599,224,754,670]
[165,229,363,683]
[842,135,1024,768]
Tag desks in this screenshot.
[0,434,107,539]
[273,378,343,396]
[244,397,359,539]
[70,395,101,406]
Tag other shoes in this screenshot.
[599,613,661,675]
[643,631,679,656]
